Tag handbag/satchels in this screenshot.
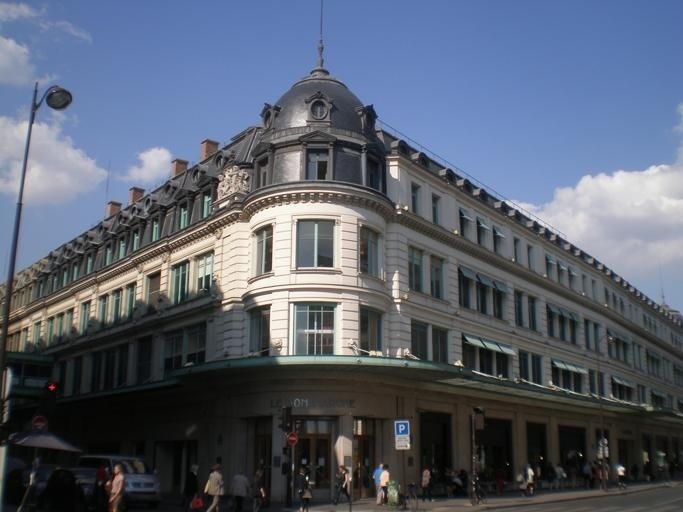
[189,497,203,510]
[300,487,313,500]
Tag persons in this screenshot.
[372,463,383,504]
[203,464,226,511]
[295,467,312,511]
[228,468,252,512]
[103,463,125,511]
[515,456,629,497]
[251,457,270,512]
[182,463,204,511]
[642,460,651,483]
[379,463,390,506]
[331,465,351,505]
[420,463,433,502]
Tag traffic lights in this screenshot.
[278,406,292,432]
[46,382,57,407]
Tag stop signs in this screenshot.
[286,433,299,446]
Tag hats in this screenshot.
[209,460,225,471]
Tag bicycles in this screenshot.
[399,485,418,510]
[467,477,487,506]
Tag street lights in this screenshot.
[1,82,72,376]
[596,333,617,491]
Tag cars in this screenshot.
[6,454,162,510]
